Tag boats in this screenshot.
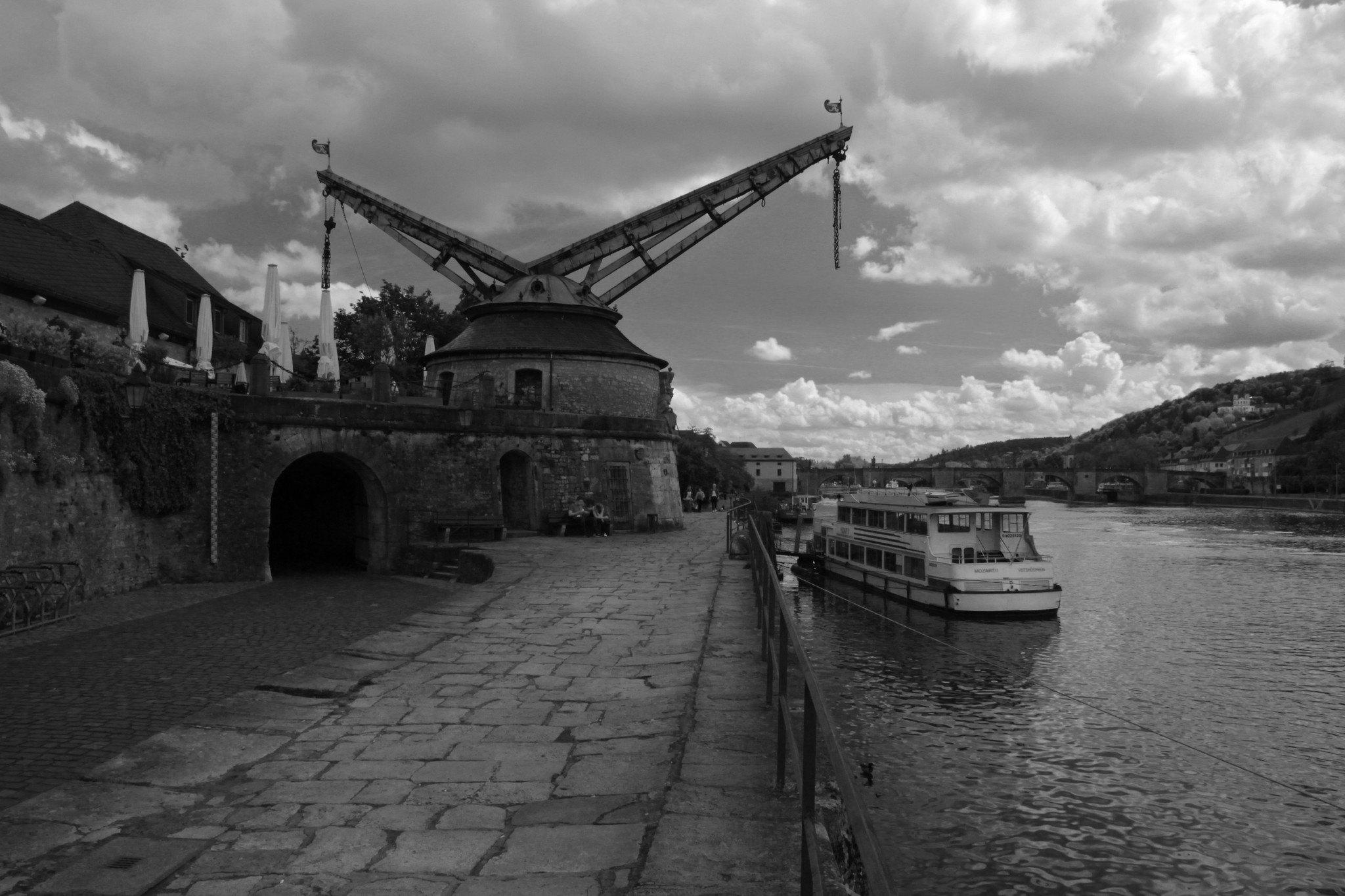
[805,479,1064,621]
[1096,481,1135,502]
[772,494,824,523]
[820,483,862,494]
[1024,476,1069,498]
[924,491,962,505]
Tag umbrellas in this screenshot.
[125,269,150,373]
[317,290,340,382]
[381,314,396,366]
[235,359,247,384]
[423,334,436,392]
[259,264,294,392]
[196,294,216,380]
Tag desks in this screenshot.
[176,376,215,388]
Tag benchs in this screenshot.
[546,511,615,537]
[433,514,506,542]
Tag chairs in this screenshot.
[186,368,326,391]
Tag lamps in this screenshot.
[31,294,47,308]
[157,330,170,341]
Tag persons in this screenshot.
[782,502,785,506]
[392,381,399,402]
[569,497,608,537]
[683,484,719,513]
[793,502,802,510]
[731,489,738,496]
[821,481,836,486]
[498,381,536,403]
[720,492,727,500]
